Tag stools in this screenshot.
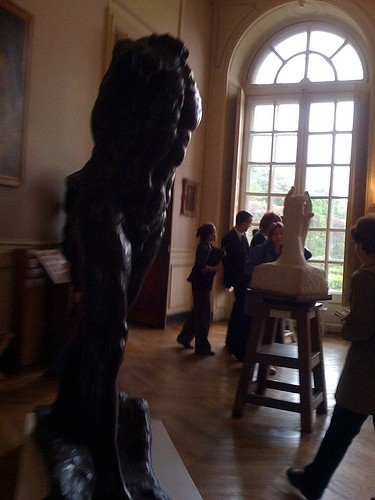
[231,289,318,433]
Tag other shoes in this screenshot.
[286,467,324,500]
[176,336,193,349]
[195,349,216,356]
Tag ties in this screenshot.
[241,235,248,250]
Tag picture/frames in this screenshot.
[0,0,35,190]
[180,178,199,217]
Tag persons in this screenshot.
[250,213,282,246]
[176,221,227,357]
[276,184,314,263]
[246,223,311,374]
[284,215,375,500]
[220,210,253,357]
[57,33,203,500]
